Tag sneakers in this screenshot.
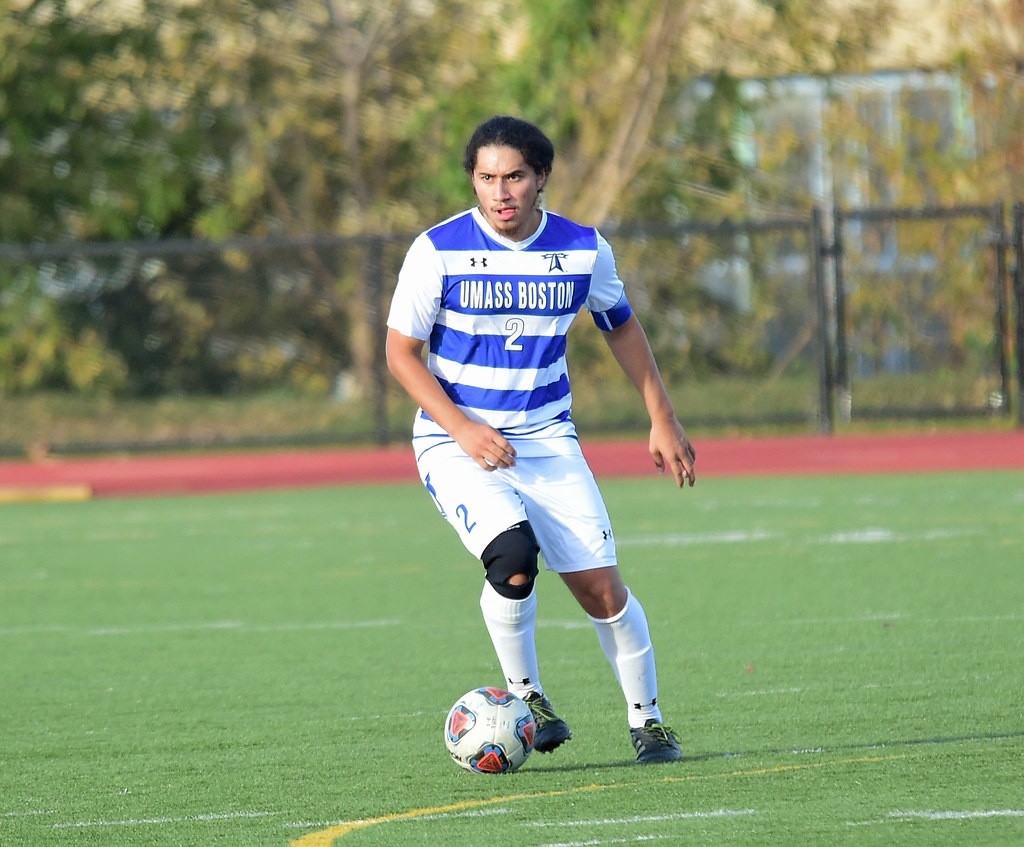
[522,690,573,754]
[630,718,684,764]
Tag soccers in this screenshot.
[443,685,537,776]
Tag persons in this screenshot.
[386,115,696,766]
